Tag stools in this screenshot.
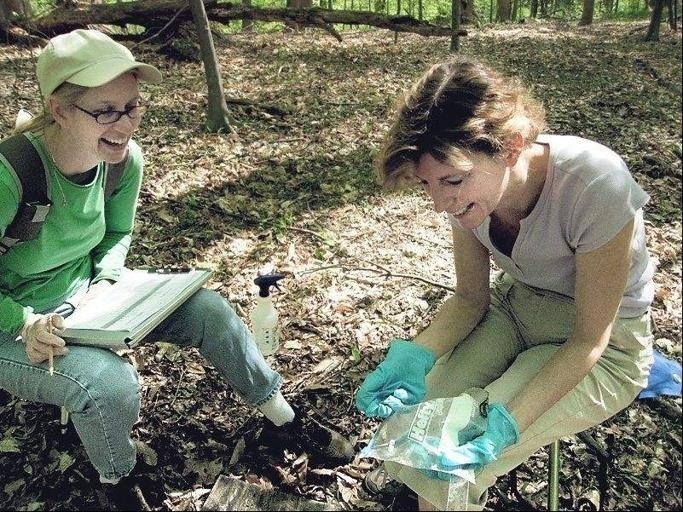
[550,431,614,510]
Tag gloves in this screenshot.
[24,313,68,365]
[354,339,437,417]
[417,402,521,482]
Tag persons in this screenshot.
[0,28,357,510]
[356,58,656,511]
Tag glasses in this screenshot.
[72,102,150,125]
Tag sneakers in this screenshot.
[270,408,356,466]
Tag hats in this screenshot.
[36,28,163,97]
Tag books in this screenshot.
[45,266,214,350]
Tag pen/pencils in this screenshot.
[48,317,54,377]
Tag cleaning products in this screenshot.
[251,271,287,357]
